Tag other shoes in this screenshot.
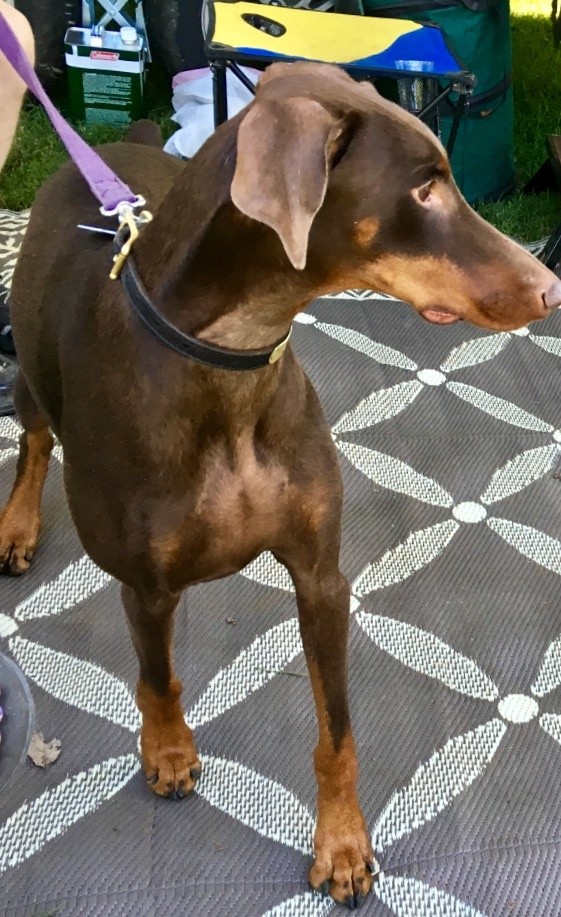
[0,319,17,415]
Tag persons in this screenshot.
[0,0,35,175]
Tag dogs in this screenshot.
[1,59,561,909]
